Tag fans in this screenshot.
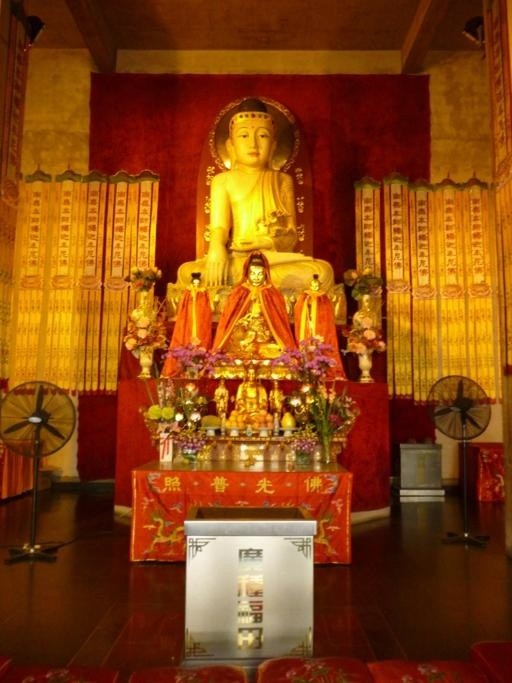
[423,373,494,549]
[1,379,79,568]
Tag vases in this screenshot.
[354,352,377,383]
[128,289,159,322]
[352,294,379,331]
[136,347,156,380]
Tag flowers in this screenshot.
[270,338,336,379]
[341,317,388,357]
[164,343,226,373]
[124,265,162,293]
[343,265,384,304]
[290,384,359,464]
[140,380,213,460]
[121,307,169,353]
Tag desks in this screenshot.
[128,457,356,568]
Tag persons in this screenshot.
[300,277,326,352]
[352,294,382,345]
[215,379,230,420]
[177,96,332,294]
[185,273,205,346]
[234,365,268,418]
[269,380,284,415]
[216,257,287,354]
